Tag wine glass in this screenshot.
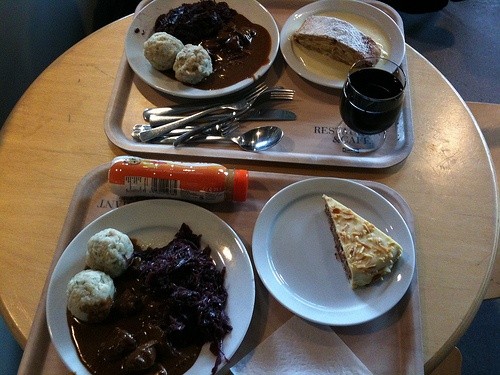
[336,57,407,152]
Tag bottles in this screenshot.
[107,157,249,204]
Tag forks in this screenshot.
[129,82,295,142]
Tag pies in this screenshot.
[321,193,403,288]
[295,15,381,69]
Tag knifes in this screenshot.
[148,107,297,127]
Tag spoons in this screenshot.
[158,126,284,152]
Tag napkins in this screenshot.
[229,314,374,375]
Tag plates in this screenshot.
[45,199,256,375]
[124,0,279,98]
[280,0,405,88]
[251,178,416,326]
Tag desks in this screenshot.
[0,13,500,375]
